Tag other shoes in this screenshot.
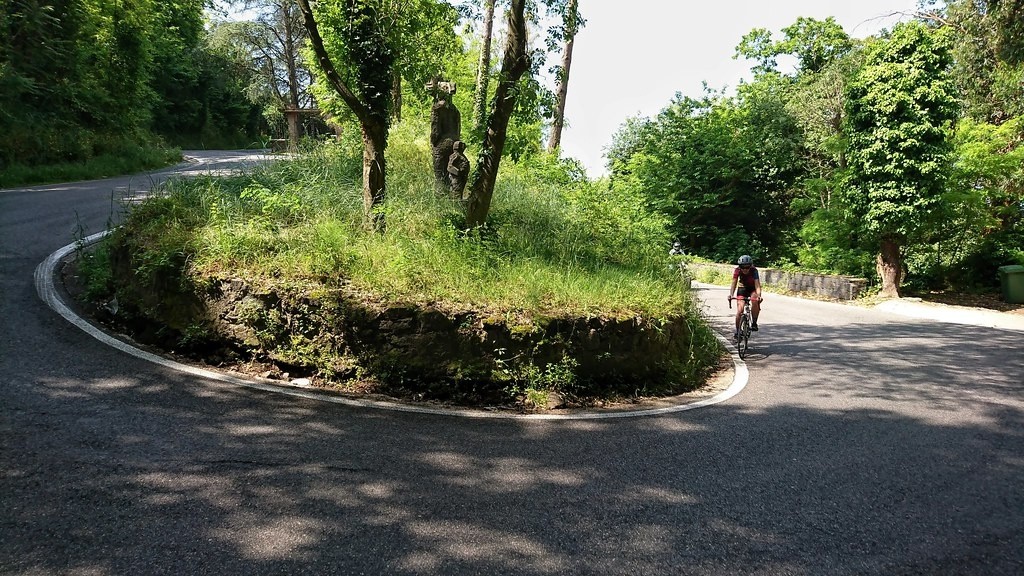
[751,323,758,331]
[733,333,741,343]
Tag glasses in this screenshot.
[739,265,751,269]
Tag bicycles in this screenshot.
[727,295,764,359]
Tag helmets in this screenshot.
[673,242,681,250]
[738,254,753,266]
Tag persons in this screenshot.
[669,242,689,270]
[728,255,763,340]
